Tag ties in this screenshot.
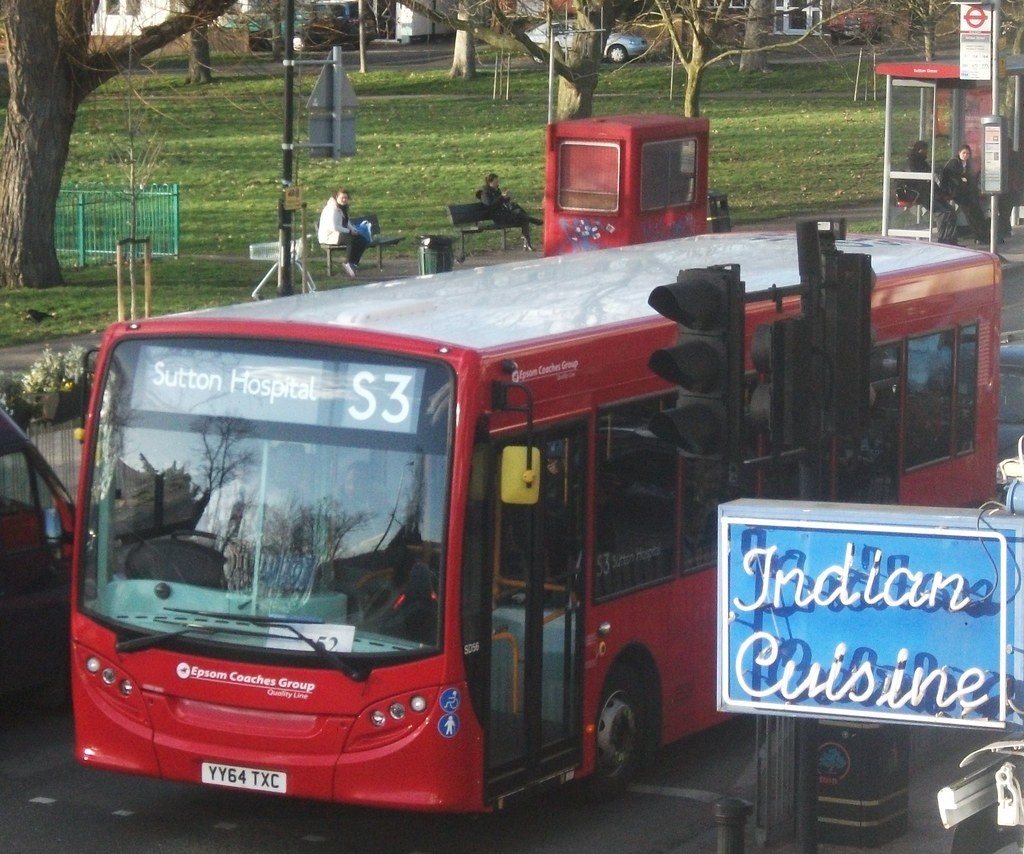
[221,487,246,558]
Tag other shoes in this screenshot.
[530,217,544,225]
[343,261,358,279]
[523,242,534,251]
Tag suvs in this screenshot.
[292,0,378,53]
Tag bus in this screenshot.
[61,222,1001,813]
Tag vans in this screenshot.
[1,411,79,709]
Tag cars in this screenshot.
[526,20,647,64]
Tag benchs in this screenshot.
[908,193,990,229]
[313,214,406,277]
[447,203,520,255]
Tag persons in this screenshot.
[943,144,990,245]
[901,141,964,246]
[341,462,392,520]
[480,174,543,251]
[543,437,572,475]
[192,445,298,583]
[318,188,366,277]
[998,139,1024,236]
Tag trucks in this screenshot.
[829,0,906,44]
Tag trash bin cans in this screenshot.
[416,234,455,276]
[705,190,732,233]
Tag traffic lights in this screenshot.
[752,320,813,445]
[645,263,747,464]
